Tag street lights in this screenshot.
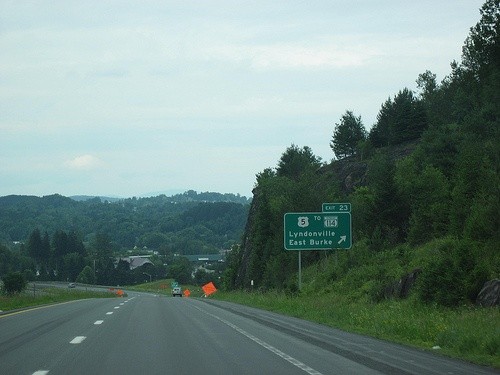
[142,272,152,293]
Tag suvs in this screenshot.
[68,283,75,288]
[173,287,182,297]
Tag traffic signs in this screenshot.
[284,211,354,250]
